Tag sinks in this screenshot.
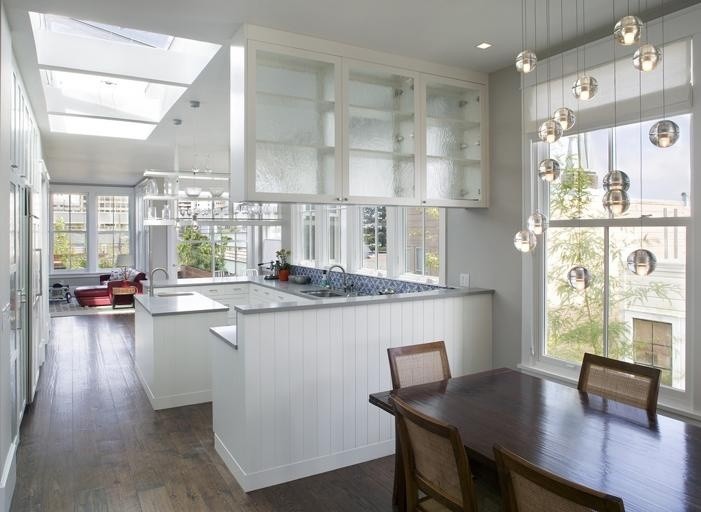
[158,293,193,297]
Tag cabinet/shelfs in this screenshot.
[152,284,311,325]
[230,23,490,210]
[142,171,284,226]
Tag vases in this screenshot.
[277,270,289,281]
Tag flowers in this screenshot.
[276,249,291,274]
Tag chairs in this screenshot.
[388,341,451,389]
[110,285,135,310]
[578,353,661,413]
[579,390,659,436]
[49,283,72,303]
[389,393,503,512]
[493,441,626,512]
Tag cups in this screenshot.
[147,207,156,220]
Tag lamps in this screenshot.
[513,0,680,289]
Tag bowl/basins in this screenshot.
[379,288,395,295]
[288,275,311,284]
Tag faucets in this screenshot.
[300,290,342,298]
[326,266,347,293]
[150,268,168,296]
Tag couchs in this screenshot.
[74,269,147,307]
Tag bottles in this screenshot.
[320,270,327,287]
[265,260,280,279]
[162,204,170,219]
